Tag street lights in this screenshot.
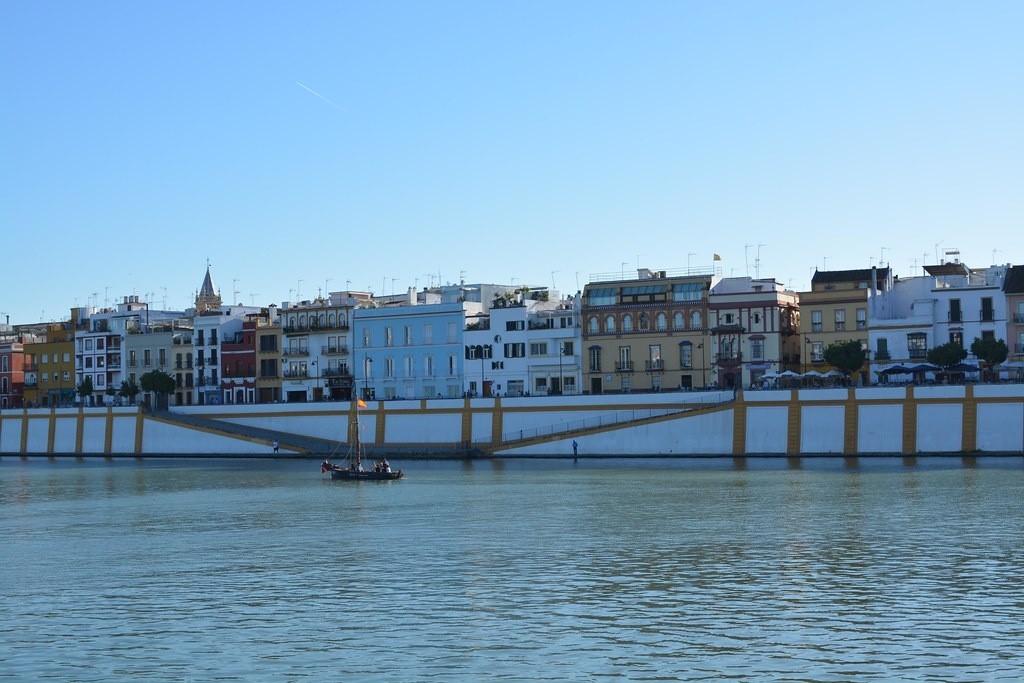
[311,355,320,401]
[803,332,812,390]
[481,345,490,398]
[364,353,373,400]
[696,338,706,391]
[559,343,566,396]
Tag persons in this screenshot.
[520,430,523,439]
[350,457,392,473]
[0,399,117,409]
[209,360,1024,403]
[572,440,578,455]
[273,440,279,453]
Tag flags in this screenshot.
[357,398,366,409]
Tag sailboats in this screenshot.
[322,394,405,481]
